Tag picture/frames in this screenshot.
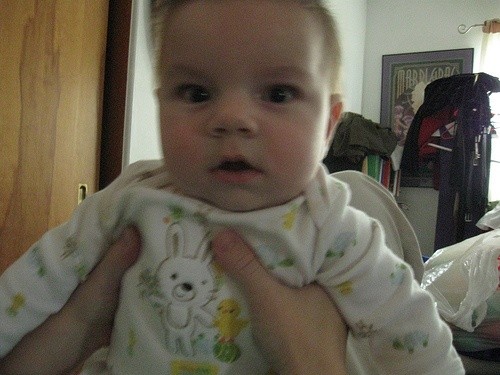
[380,46,476,188]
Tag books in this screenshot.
[360,148,402,198]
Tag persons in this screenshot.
[0,1,471,375]
[5,228,353,374]
